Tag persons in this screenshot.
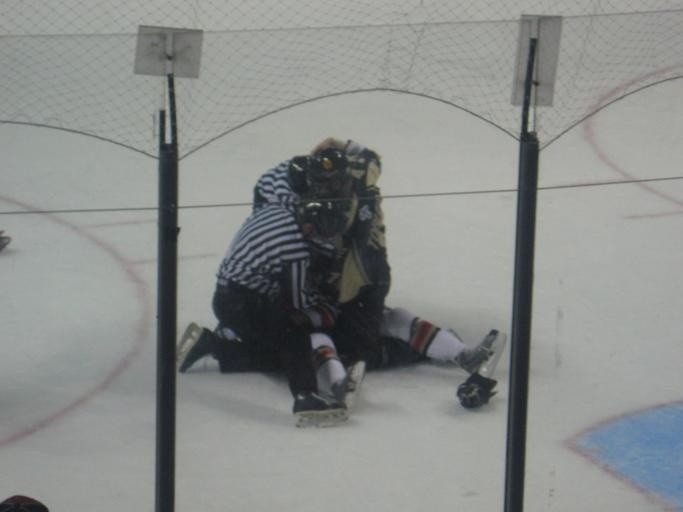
[215,139,506,408]
[178,200,351,426]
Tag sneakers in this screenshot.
[292,360,367,417]
[177,322,214,373]
[454,330,502,379]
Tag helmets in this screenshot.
[288,148,351,238]
